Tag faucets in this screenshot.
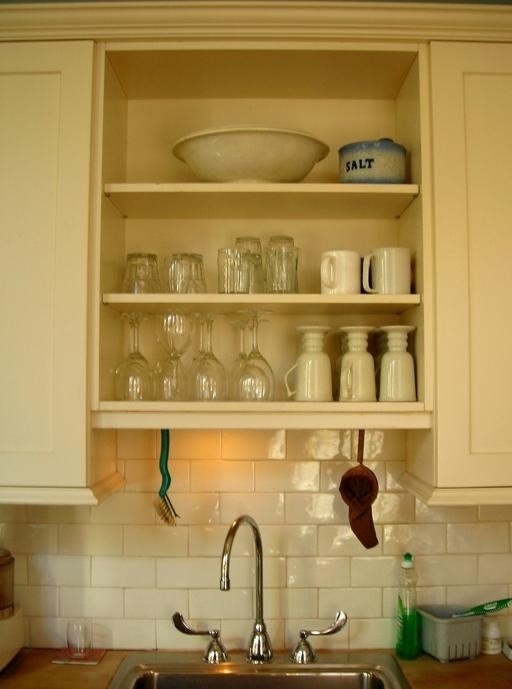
[220,515,274,661]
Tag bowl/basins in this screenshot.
[172,127,330,182]
[338,138,406,184]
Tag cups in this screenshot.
[338,326,377,402]
[67,624,91,659]
[219,236,298,294]
[165,253,206,292]
[380,326,416,403]
[363,247,411,294]
[320,250,361,296]
[284,326,333,402]
[121,253,161,293]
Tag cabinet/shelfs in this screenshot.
[89,38,434,430]
[1,40,126,506]
[396,40,512,504]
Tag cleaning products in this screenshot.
[395,552,419,658]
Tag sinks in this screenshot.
[106,651,412,689]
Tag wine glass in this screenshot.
[111,311,155,402]
[158,309,192,400]
[230,310,274,400]
[192,311,228,400]
